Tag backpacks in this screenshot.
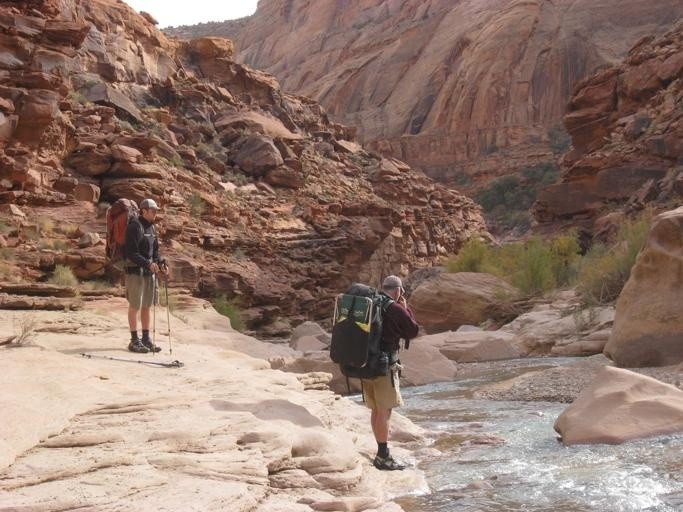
[104,197,146,273]
[329,280,396,380]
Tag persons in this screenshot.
[124,199,168,353]
[361,275,418,470]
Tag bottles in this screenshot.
[380,352,388,377]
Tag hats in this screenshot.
[383,275,406,295]
[140,200,160,211]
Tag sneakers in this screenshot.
[128,340,162,354]
[373,452,405,470]
